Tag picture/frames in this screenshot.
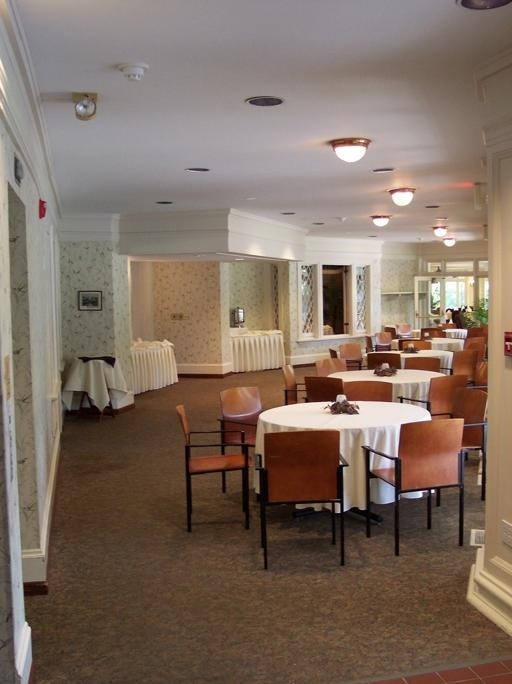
[77,291,102,311]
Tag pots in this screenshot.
[232,307,245,323]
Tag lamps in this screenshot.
[390,187,414,206]
[432,226,446,237]
[372,215,390,227]
[443,237,457,247]
[329,138,369,163]
[72,92,98,121]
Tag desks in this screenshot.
[61,356,134,424]
[131,341,178,396]
